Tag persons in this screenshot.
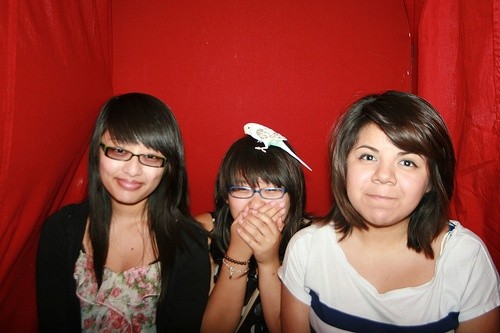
[190,122,321,333]
[275,89,500,333]
[33,90,213,333]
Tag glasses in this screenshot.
[100,141,166,168]
[227,183,287,199]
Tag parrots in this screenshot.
[244,123,313,172]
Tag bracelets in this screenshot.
[224,254,250,265]
[221,258,250,280]
